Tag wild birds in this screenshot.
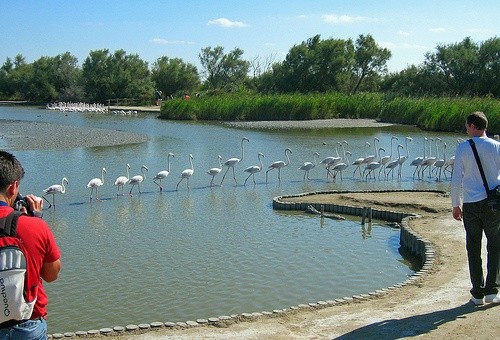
[220,137,249,186]
[46,102,138,115]
[242,152,265,187]
[266,148,293,184]
[152,152,175,192]
[43,177,68,211]
[113,164,130,197]
[319,140,351,184]
[297,152,320,180]
[352,137,464,183]
[128,165,148,196]
[176,154,194,192]
[86,167,107,201]
[206,155,222,186]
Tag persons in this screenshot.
[451,111,500,304]
[0,151,62,340]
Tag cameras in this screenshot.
[12,193,31,215]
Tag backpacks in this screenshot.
[0,209,37,327]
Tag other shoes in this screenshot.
[471,294,500,305]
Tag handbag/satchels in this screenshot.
[488,184,500,232]
